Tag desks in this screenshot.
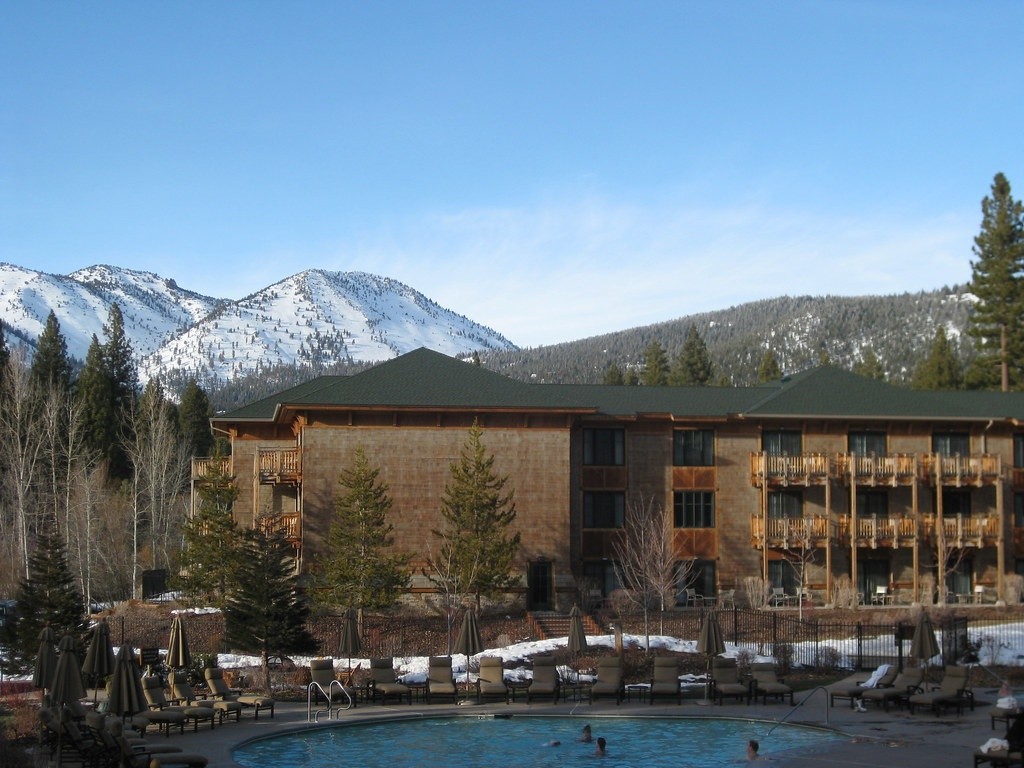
[509,685,528,703]
[563,685,584,703]
[900,694,920,712]
[628,686,648,704]
[354,687,369,705]
[407,685,427,702]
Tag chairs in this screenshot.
[310,659,357,711]
[650,657,681,705]
[709,658,750,706]
[38,681,210,768]
[168,673,241,726]
[527,656,560,704]
[367,657,412,706]
[589,656,625,705]
[205,668,276,720]
[477,657,509,704]
[141,675,215,732]
[426,657,458,705]
[750,663,793,706]
[831,665,1024,768]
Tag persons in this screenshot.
[594,737,606,754]
[580,725,593,742]
[746,740,759,759]
[998,680,1014,698]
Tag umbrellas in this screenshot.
[164,613,191,706]
[909,605,941,695]
[48,633,88,768]
[567,602,588,671]
[30,620,59,745]
[693,604,726,705]
[108,638,150,768]
[80,614,116,712]
[453,600,485,706]
[337,602,361,688]
